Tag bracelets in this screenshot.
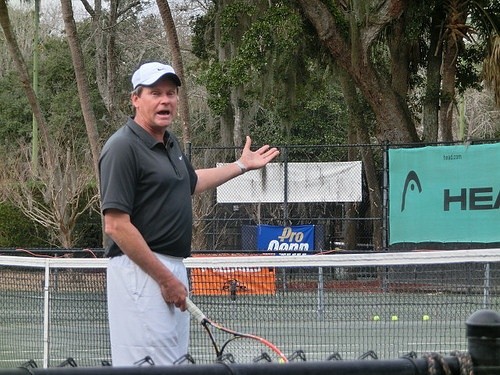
[234,160,247,173]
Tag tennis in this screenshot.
[373,315,380,321]
[392,316,397,320]
[422,315,429,320]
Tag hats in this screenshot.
[130,62,181,89]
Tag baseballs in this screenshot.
[185,298,288,364]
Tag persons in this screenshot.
[98,62,280,364]
